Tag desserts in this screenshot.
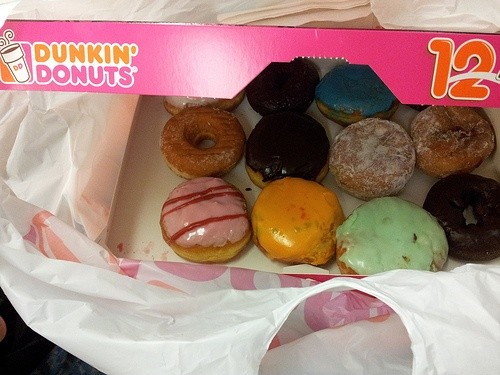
[314,64,395,126]
[335,197,448,275]
[163,88,245,116]
[246,58,321,114]
[329,117,415,200]
[160,177,251,263]
[245,110,330,189]
[250,176,345,266]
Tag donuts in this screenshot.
[161,105,244,180]
[423,171,500,265]
[408,102,496,178]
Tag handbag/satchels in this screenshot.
[1,0,499,375]
